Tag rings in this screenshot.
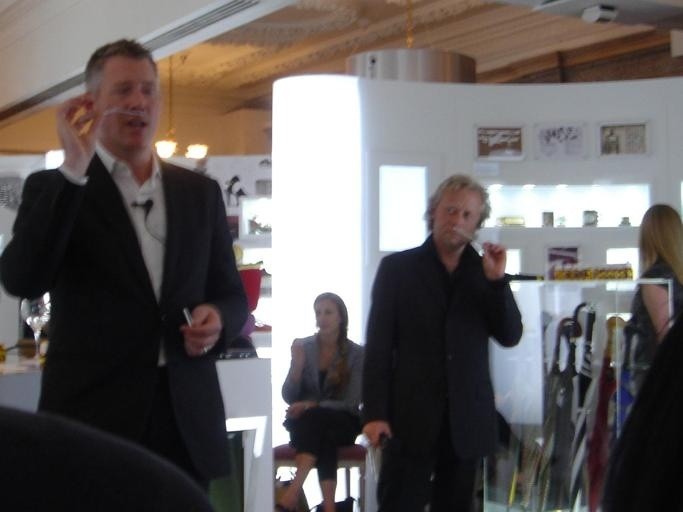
[204,347,207,354]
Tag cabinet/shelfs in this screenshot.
[208,157,272,348]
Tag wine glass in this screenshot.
[20,292,52,369]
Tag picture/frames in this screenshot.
[474,120,652,163]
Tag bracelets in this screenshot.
[304,400,309,412]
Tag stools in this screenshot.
[271,441,366,511]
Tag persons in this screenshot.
[274,292,365,512]
[0,39,248,494]
[361,174,523,512]
[631,204,683,392]
[602,308,683,512]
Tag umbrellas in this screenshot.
[508,300,637,512]
